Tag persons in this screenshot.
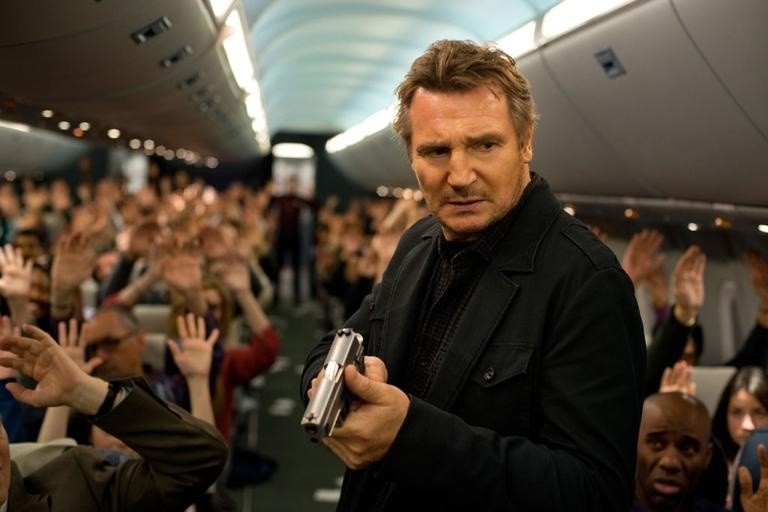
[266,174,768,512]
[298,38,647,511]
[1,157,277,511]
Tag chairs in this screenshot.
[688,364,738,419]
[144,334,168,372]
[133,303,175,330]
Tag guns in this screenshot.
[301,328,365,443]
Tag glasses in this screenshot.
[85,327,141,358]
